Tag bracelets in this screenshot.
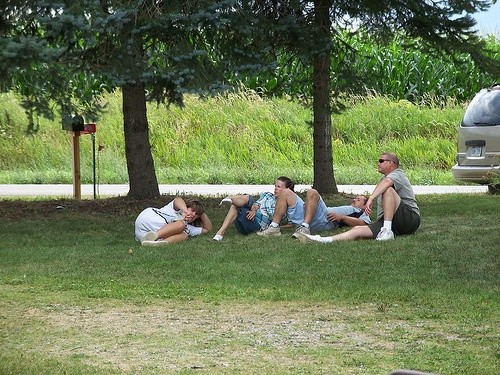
[369,196,375,201]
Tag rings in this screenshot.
[333,217,334,218]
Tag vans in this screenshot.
[451,85,500,194]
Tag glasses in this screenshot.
[379,159,391,164]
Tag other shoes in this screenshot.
[298,234,324,244]
[141,232,158,241]
[212,235,224,241]
[374,228,395,240]
[256,225,282,236]
[142,241,169,247]
[292,226,311,238]
[219,199,232,209]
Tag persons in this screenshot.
[257,189,368,239]
[135,197,213,246]
[299,152,420,244]
[212,177,296,241]
